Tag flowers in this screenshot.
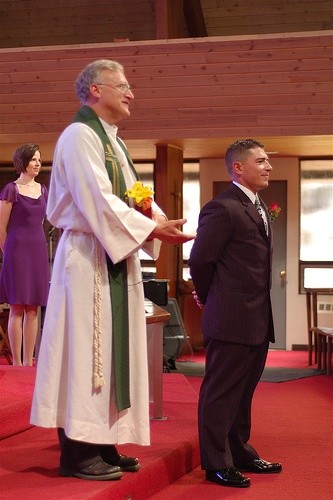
[266,201,285,224]
[124,180,155,219]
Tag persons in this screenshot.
[0,143,52,367]
[188,137,284,488]
[29,60,197,481]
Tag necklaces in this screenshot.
[17,177,36,187]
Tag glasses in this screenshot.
[96,84,132,92]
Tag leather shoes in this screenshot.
[99,447,141,471]
[60,455,123,480]
[233,457,282,473]
[206,466,250,487]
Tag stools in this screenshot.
[0,306,13,365]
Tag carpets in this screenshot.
[166,360,331,384]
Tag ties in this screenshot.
[255,200,267,232]
